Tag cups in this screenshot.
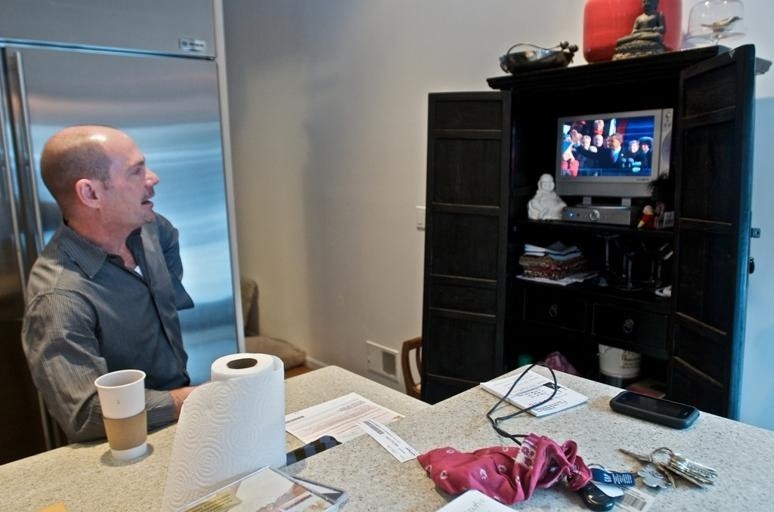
[92,369,148,463]
[595,336,646,383]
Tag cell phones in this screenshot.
[610,390,699,430]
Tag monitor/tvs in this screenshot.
[554,108,673,206]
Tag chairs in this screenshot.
[400,335,421,398]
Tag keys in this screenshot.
[616,442,718,492]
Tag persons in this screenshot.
[561,118,654,177]
[15,123,203,443]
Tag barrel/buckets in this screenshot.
[598,345,641,379]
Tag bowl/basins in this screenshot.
[494,40,579,78]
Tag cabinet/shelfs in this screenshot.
[420,45,757,416]
